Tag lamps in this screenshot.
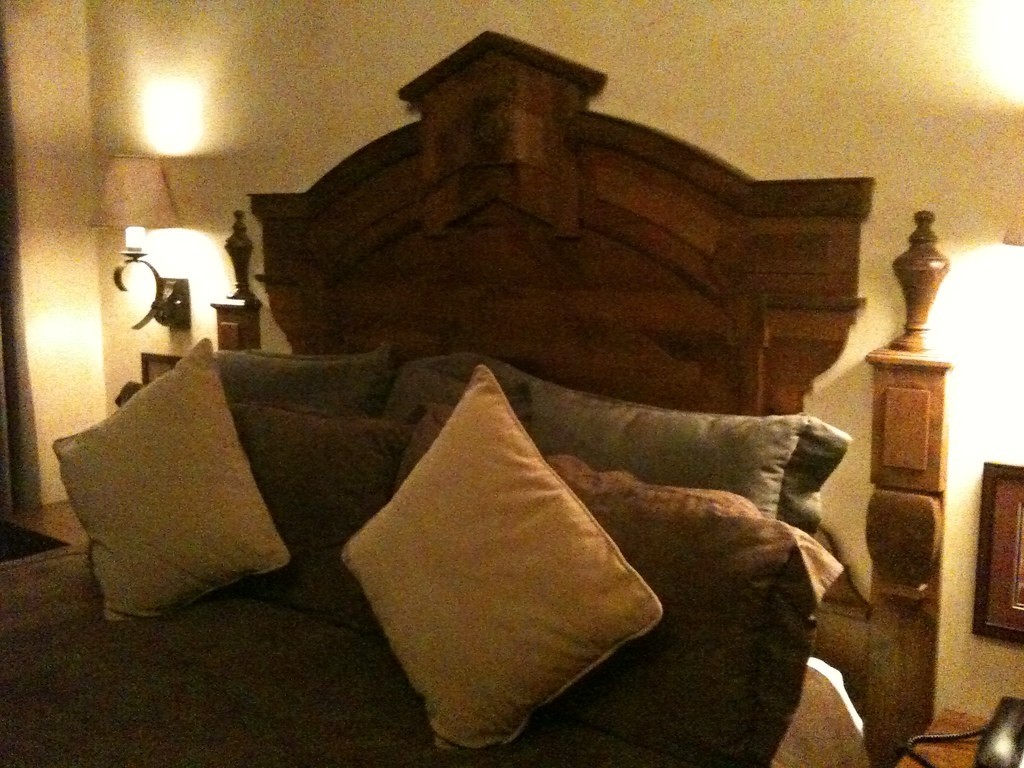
[135,71,207,154]
[91,154,191,332]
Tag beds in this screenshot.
[0,31,951,768]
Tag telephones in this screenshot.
[972,696,1024,768]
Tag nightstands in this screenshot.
[6,498,90,562]
[897,710,990,767]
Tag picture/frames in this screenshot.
[973,463,1024,645]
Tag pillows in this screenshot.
[388,351,851,537]
[392,408,845,768]
[52,339,290,620]
[214,344,394,417]
[341,363,664,749]
[116,381,417,635]
